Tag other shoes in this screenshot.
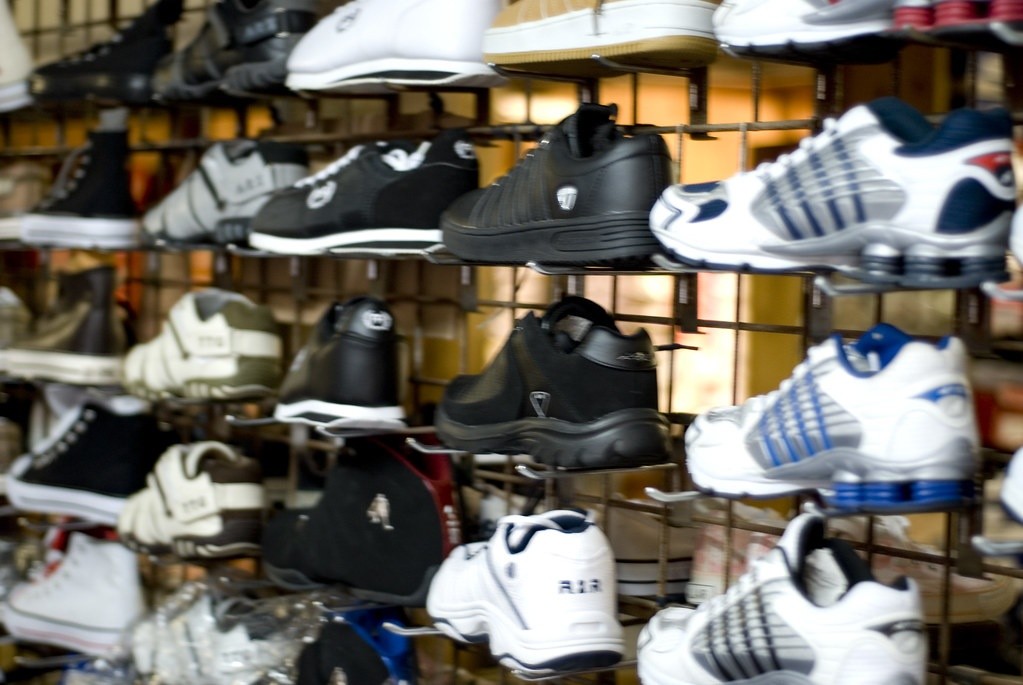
[713,0,1023,67]
[286,0,507,86]
[483,0,716,79]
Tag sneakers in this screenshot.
[635,513,927,685]
[115,286,284,404]
[0,385,168,527]
[650,95,1019,289]
[435,297,670,468]
[440,99,671,264]
[141,135,313,251]
[147,0,325,102]
[134,569,420,685]
[0,516,147,660]
[260,297,407,433]
[117,440,265,562]
[248,139,482,252]
[262,432,469,605]
[684,322,982,517]
[0,0,185,384]
[422,503,624,678]
